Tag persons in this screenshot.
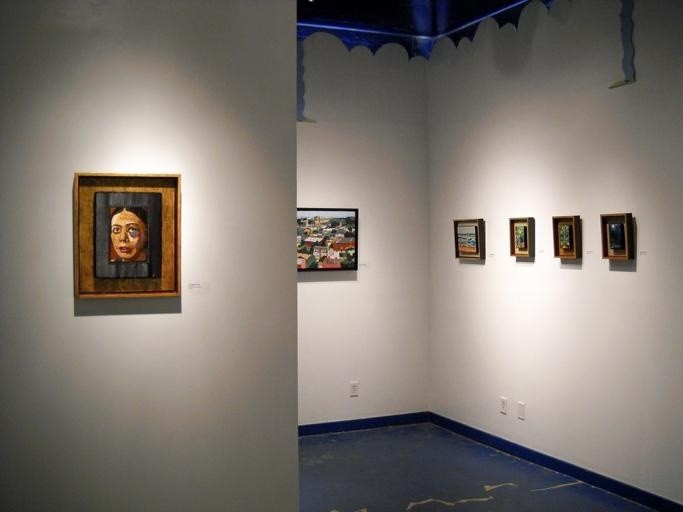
[111,208,146,262]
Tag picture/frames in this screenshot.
[71,171,182,300]
[296,208,358,272]
[453,213,634,261]
